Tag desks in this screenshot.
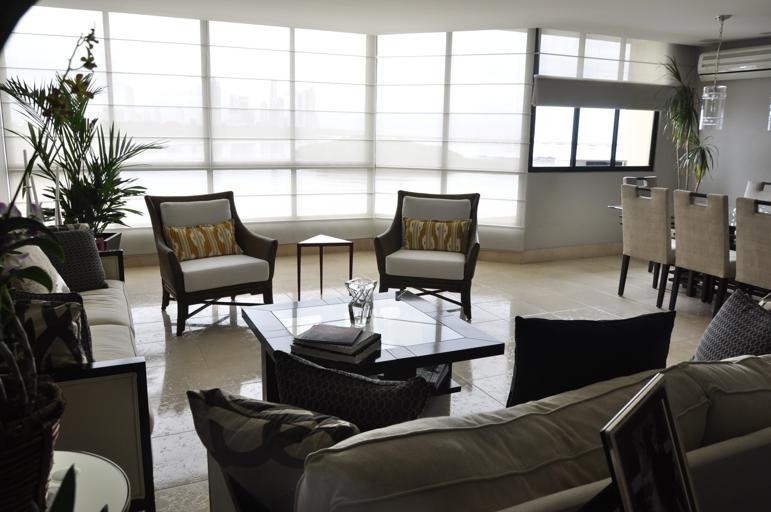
[1,447,132,512]
[295,233,358,299]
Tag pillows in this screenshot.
[504,304,676,407]
[163,219,244,262]
[184,384,359,512]
[691,294,771,363]
[3,226,111,364]
[396,212,472,253]
[256,337,427,429]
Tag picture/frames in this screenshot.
[600,368,702,510]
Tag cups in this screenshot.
[347,277,378,306]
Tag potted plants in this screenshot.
[0,67,169,250]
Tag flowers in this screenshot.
[0,27,105,405]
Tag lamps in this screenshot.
[697,12,736,132]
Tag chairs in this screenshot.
[374,190,490,325]
[606,169,771,318]
[141,187,280,339]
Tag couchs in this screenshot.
[2,213,156,511]
[189,296,770,512]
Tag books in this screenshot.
[299,324,362,344]
[290,339,382,365]
[293,324,382,355]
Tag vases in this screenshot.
[0,378,66,502]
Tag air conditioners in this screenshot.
[698,43,770,85]
